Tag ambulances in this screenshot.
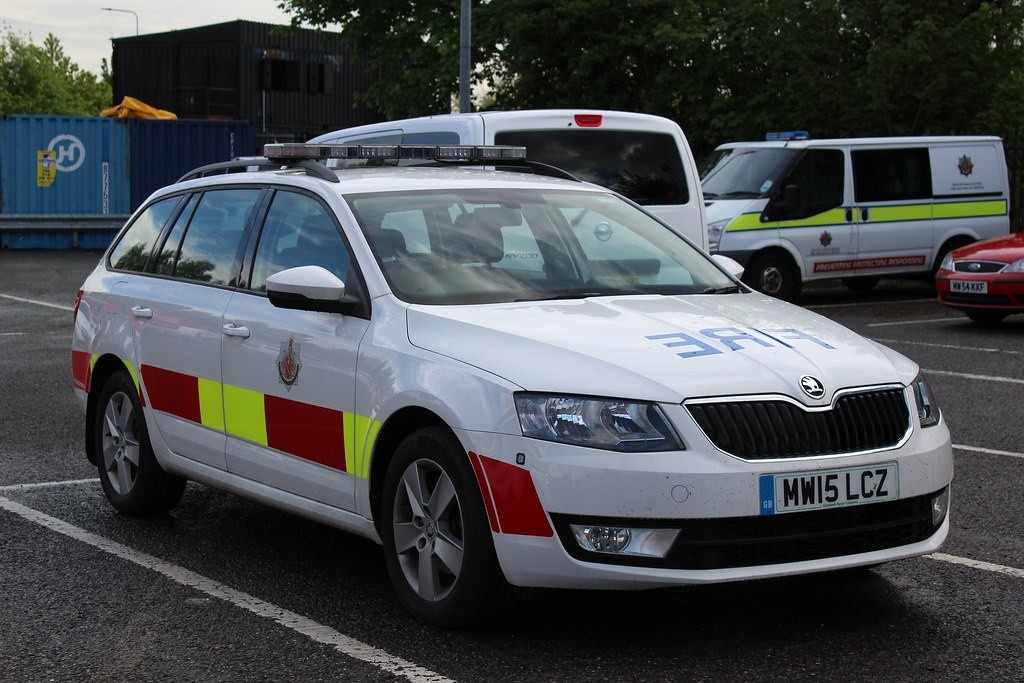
[69,142,957,635]
[699,130,1011,304]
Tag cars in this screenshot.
[934,230,1024,326]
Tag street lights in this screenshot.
[100,7,139,37]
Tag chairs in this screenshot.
[451,210,519,292]
[345,228,404,301]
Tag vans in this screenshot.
[304,107,710,288]
